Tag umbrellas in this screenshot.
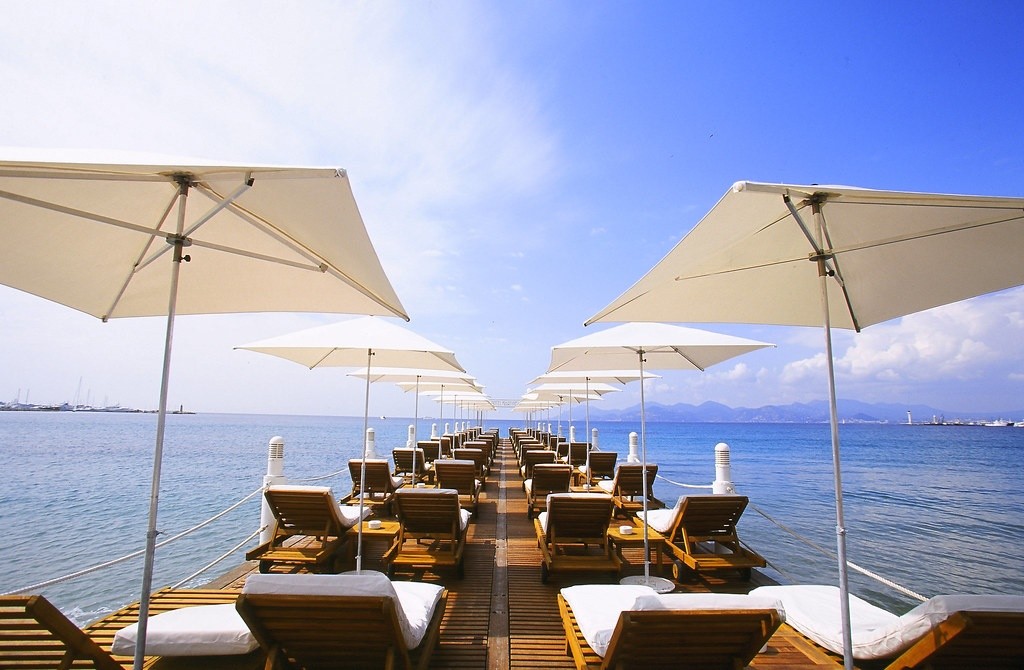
[584,181,1024,670]
[346,365,664,494]
[232,315,468,576]
[0,163,411,670]
[547,321,777,585]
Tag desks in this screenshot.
[570,487,604,493]
[572,468,580,487]
[607,527,665,575]
[402,485,436,489]
[345,520,400,574]
[749,621,844,670]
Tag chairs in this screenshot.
[485,431,497,450]
[470,428,477,439]
[392,447,432,483]
[490,428,500,440]
[382,488,468,579]
[434,459,481,520]
[457,432,466,445]
[340,458,404,515]
[431,437,452,458]
[474,439,493,462]
[476,427,482,435]
[234,573,449,670]
[417,441,447,463]
[0,585,241,670]
[246,484,369,573]
[489,429,499,445]
[443,434,460,448]
[468,430,474,440]
[509,427,1024,670]
[478,435,496,458]
[453,448,486,491]
[463,442,491,475]
